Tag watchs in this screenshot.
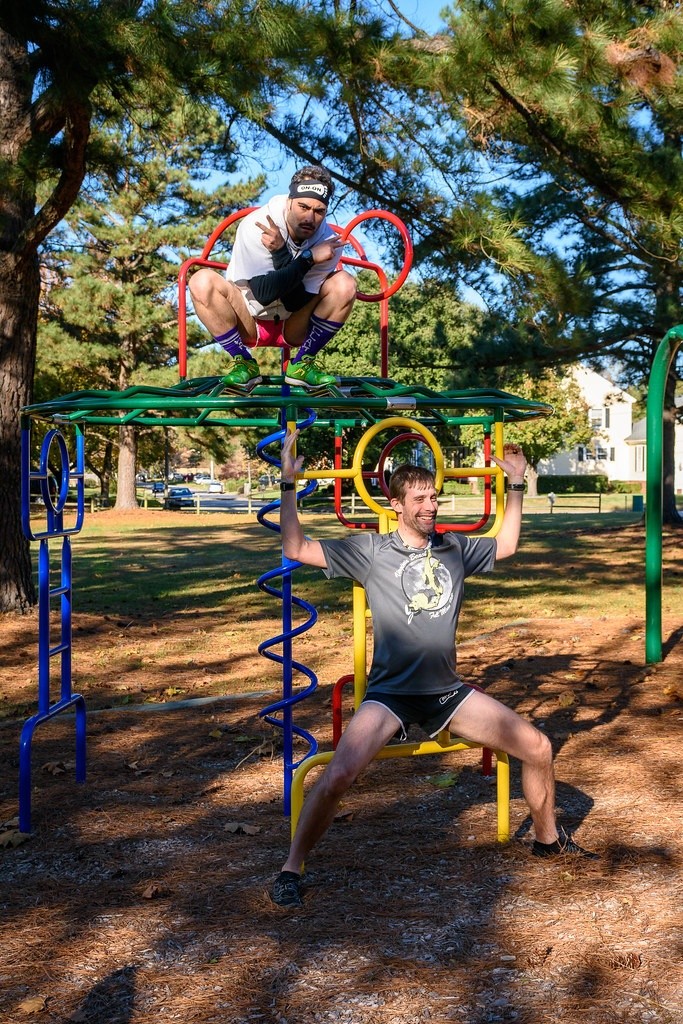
[302,249,315,267]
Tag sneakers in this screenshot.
[272,871,301,908]
[221,355,262,387]
[532,836,601,860]
[285,354,336,389]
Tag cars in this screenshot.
[154,472,224,494]
[164,487,195,510]
[152,481,165,493]
[259,475,282,486]
[137,475,145,481]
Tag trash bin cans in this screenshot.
[633,495,643,512]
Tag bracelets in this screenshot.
[280,482,297,491]
[505,483,526,491]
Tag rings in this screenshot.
[513,451,517,454]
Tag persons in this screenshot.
[187,164,358,393]
[270,422,602,906]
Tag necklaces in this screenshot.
[273,222,301,325]
